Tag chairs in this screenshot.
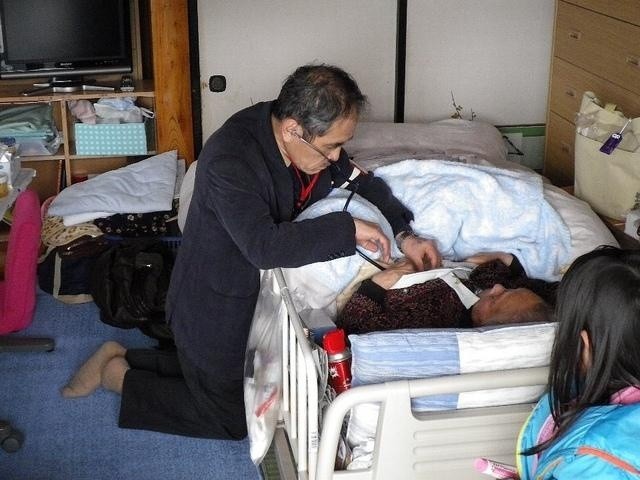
[1,187,42,337]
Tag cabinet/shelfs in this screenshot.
[543,0,640,185]
[0,79,155,193]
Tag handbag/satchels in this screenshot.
[36,237,172,338]
[573,91,639,223]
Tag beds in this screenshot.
[282,119,625,479]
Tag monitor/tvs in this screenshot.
[0,1,133,96]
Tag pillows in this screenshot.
[345,319,561,411]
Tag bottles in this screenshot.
[321,328,353,396]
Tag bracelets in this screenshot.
[396,231,419,253]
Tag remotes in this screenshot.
[120,76,134,92]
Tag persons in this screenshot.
[335,247,559,334]
[514,242,640,479]
[61,60,444,441]
